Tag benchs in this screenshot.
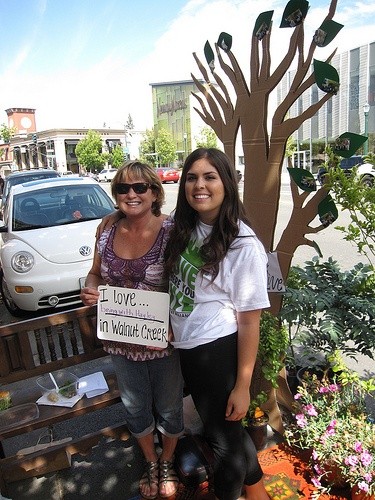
[0,304,136,499]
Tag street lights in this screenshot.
[183,133,187,156]
[362,100,370,156]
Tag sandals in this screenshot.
[159,456,180,497]
[139,456,159,499]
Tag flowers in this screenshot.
[241,369,375,500]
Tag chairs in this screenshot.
[58,193,88,215]
[19,198,48,226]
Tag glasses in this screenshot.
[116,182,154,194]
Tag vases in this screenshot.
[323,463,349,489]
[351,484,375,500]
[248,413,269,450]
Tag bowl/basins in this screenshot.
[35,370,80,404]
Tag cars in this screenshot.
[60,171,79,177]
[97,168,119,182]
[0,176,121,316]
[87,172,97,181]
[155,167,179,184]
[300,158,329,174]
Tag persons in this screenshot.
[78,166,191,499]
[94,148,271,500]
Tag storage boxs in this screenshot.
[32,367,82,404]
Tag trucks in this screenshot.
[0,160,53,182]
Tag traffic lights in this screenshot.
[33,135,37,143]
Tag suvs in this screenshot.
[316,155,375,192]
[0,166,61,213]
